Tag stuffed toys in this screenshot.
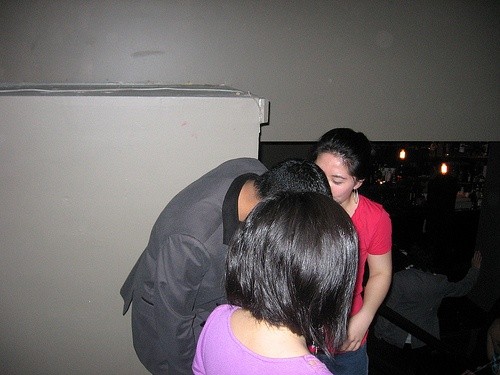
[486,318,500,375]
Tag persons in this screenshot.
[191,189,360,375]
[368,232,483,374]
[120,157,334,375]
[308,128,393,374]
[358,157,459,248]
